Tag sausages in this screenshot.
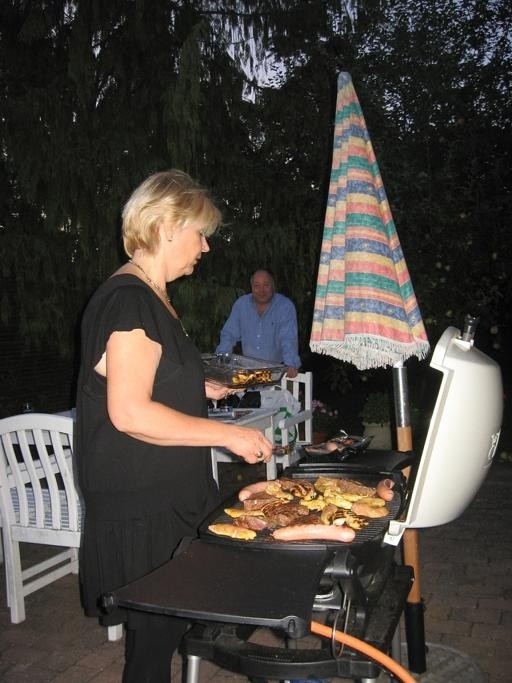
[376,478,395,501]
[273,524,355,543]
[237,481,271,502]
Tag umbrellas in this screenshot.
[309,67,430,372]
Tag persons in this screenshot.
[73,169,274,683]
[215,268,301,378]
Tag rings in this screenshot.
[257,452,263,458]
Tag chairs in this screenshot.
[0,412,85,624]
[280,370,313,446]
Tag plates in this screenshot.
[300,443,355,463]
[200,352,287,389]
[331,436,375,454]
